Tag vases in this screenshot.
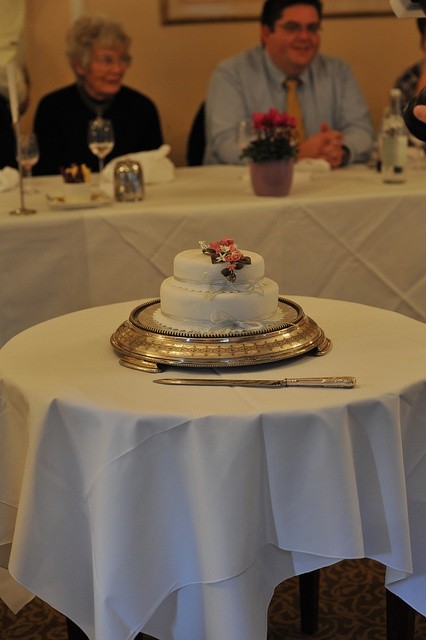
[248,157,295,197]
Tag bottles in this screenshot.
[380,89,409,183]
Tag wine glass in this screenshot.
[88,121,114,187]
[16,134,40,194]
[239,120,264,190]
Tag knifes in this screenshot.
[152,376,356,389]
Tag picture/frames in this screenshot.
[158,0,395,25]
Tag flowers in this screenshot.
[238,106,300,162]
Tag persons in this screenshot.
[32,15,164,177]
[200,0,375,170]
[1,0,32,176]
[390,0,425,151]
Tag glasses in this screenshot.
[93,53,132,64]
[274,21,323,34]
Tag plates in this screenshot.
[44,186,110,211]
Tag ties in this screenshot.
[284,77,305,147]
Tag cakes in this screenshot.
[153,237,284,335]
